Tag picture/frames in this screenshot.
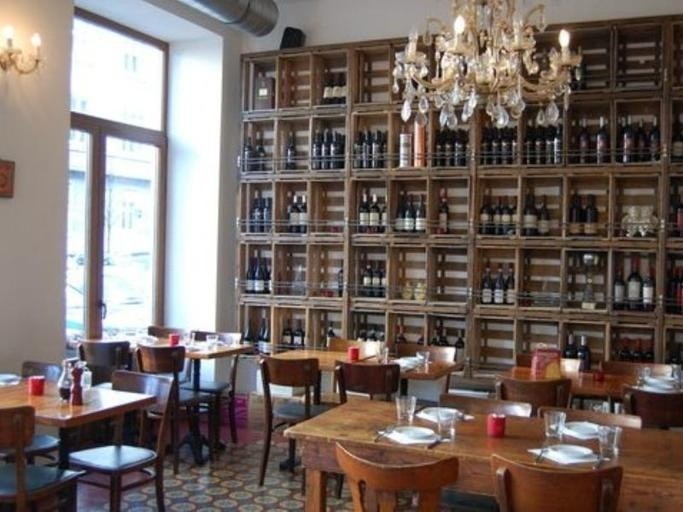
[0,160,14,199]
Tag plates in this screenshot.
[0,373,20,386]
[421,406,461,420]
[547,444,593,458]
[562,419,599,436]
[645,376,673,385]
[392,426,433,440]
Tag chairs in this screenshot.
[76,327,245,472]
[257,335,681,512]
[1,361,176,511]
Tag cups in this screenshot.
[27,375,44,395]
[393,394,416,426]
[543,411,566,438]
[169,333,195,348]
[435,411,456,443]
[595,423,623,461]
[486,413,506,436]
[206,334,218,351]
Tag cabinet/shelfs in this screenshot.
[233,11,681,394]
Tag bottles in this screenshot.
[573,46,586,90]
[481,262,515,304]
[291,264,304,296]
[56,357,92,408]
[321,74,346,104]
[337,260,343,297]
[242,313,465,363]
[359,187,683,239]
[246,113,682,169]
[580,273,596,309]
[560,333,590,372]
[244,256,270,293]
[361,259,385,297]
[247,187,309,235]
[612,253,682,314]
[401,279,425,300]
[614,331,682,362]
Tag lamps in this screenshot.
[386,1,578,134]
[0,23,43,75]
